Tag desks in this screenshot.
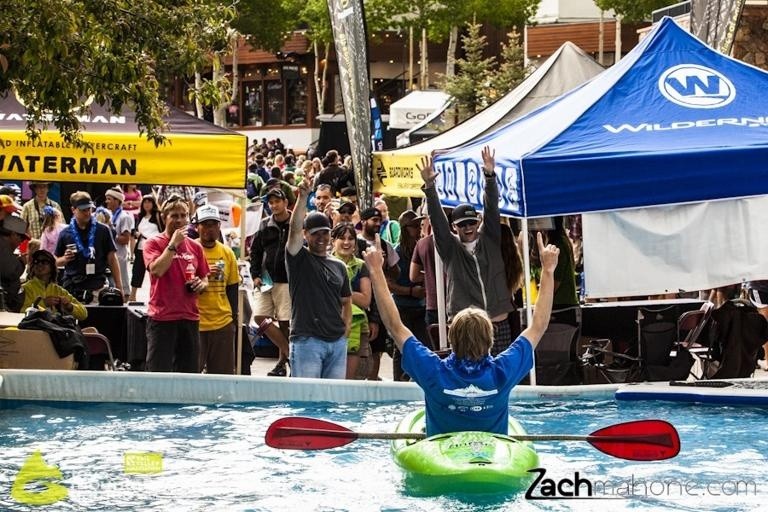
[80,301,146,372]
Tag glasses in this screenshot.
[32,258,52,265]
[457,219,478,228]
[162,194,191,212]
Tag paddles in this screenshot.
[266,415,680,461]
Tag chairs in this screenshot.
[79,331,117,372]
[428,296,766,382]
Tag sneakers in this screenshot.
[267,363,287,377]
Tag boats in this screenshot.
[391,405,541,487]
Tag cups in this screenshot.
[66,244,78,257]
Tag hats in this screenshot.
[29,180,54,190]
[32,250,55,265]
[2,215,31,241]
[452,203,482,224]
[303,210,333,235]
[0,194,23,214]
[196,204,222,223]
[261,176,427,227]
[105,187,124,203]
[70,200,97,210]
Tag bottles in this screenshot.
[185,259,197,293]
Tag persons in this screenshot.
[1,138,579,437]
